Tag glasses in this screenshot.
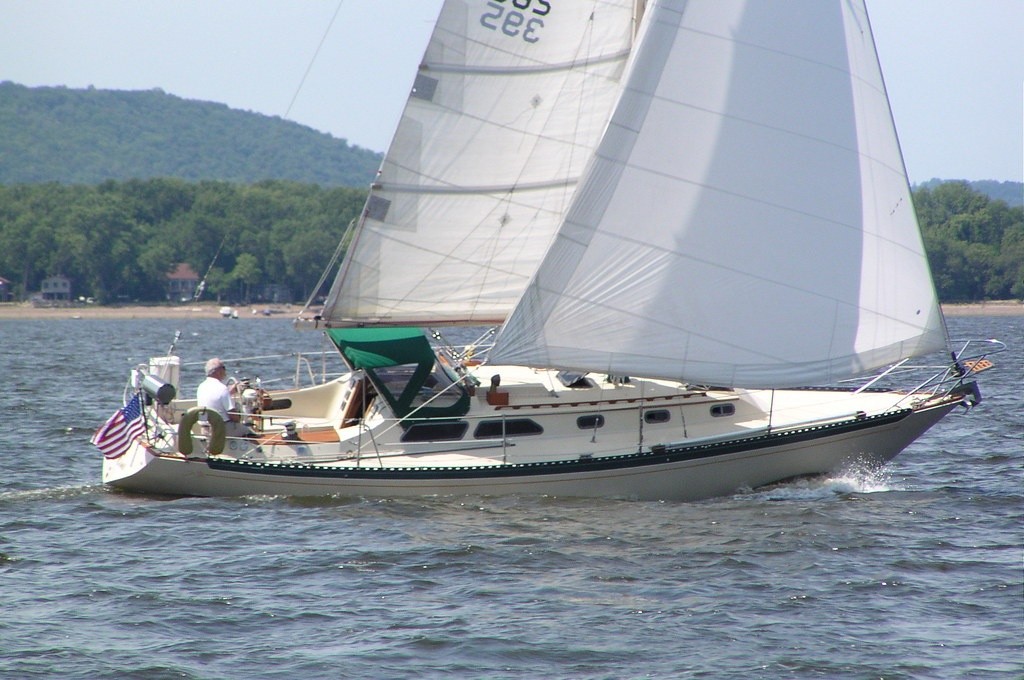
[218,365,226,370]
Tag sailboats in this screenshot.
[101,0,1007,505]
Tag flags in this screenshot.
[90,388,148,460]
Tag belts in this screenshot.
[200,420,231,427]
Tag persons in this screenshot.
[196,358,254,437]
